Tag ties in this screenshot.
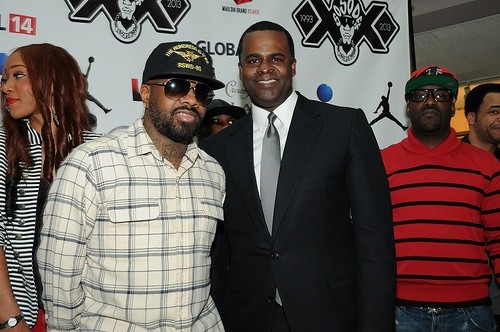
[260,111,282,235]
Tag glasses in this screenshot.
[407,88,453,102]
[145,78,215,107]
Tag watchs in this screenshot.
[0,313,24,331]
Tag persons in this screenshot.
[0,43,92,332]
[37,41,227,332]
[198,21,397,332]
[380,65,500,332]
[198,98,246,145]
[460,83,500,160]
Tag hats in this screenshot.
[404,64,458,103]
[142,41,225,91]
[203,99,246,123]
